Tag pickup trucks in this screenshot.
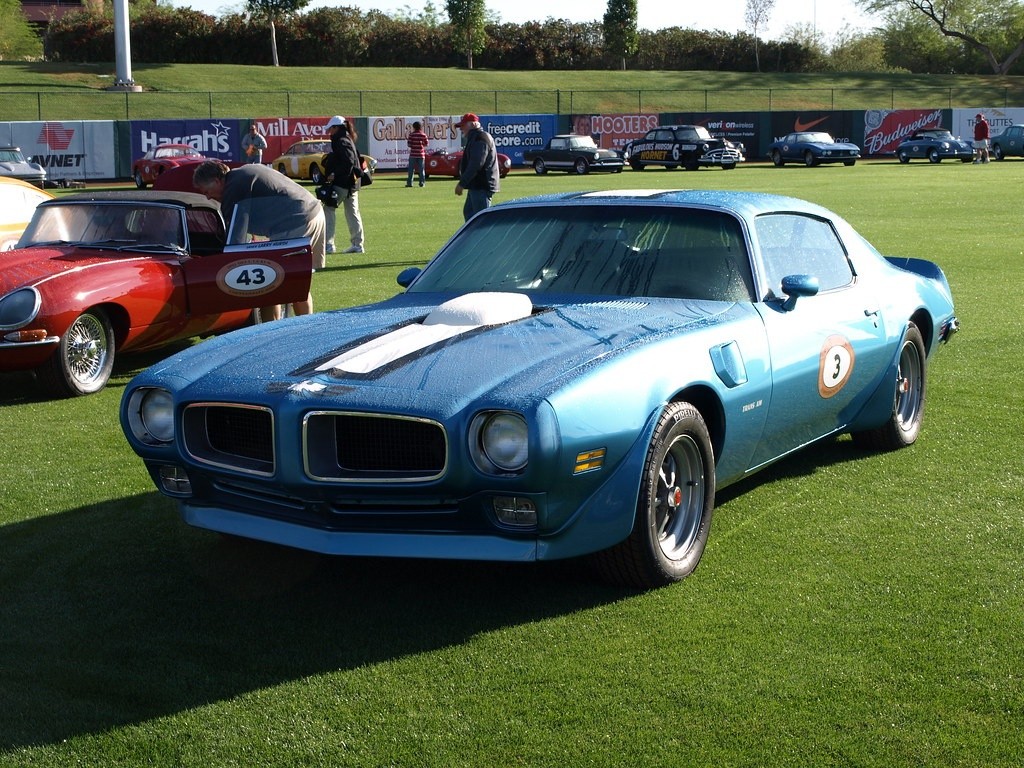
[522,134,629,176]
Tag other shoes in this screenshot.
[326,244,336,253]
[405,184,412,187]
[974,161,980,164]
[984,160,990,164]
[419,184,424,187]
[344,244,363,253]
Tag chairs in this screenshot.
[545,238,646,295]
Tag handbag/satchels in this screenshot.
[356,149,372,186]
[315,177,339,208]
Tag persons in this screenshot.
[241,124,268,164]
[574,115,600,146]
[405,122,429,187]
[453,113,501,224]
[192,160,326,324]
[323,116,363,254]
[972,112,991,164]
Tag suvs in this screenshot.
[622,124,747,171]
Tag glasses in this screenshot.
[249,129,255,131]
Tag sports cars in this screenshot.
[766,131,861,168]
[272,139,379,186]
[0,160,313,395]
[131,144,224,190]
[0,144,47,185]
[990,124,1024,160]
[896,128,978,165]
[413,145,512,181]
[120,188,961,591]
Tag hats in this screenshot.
[975,114,982,118]
[454,113,479,129]
[323,115,346,130]
